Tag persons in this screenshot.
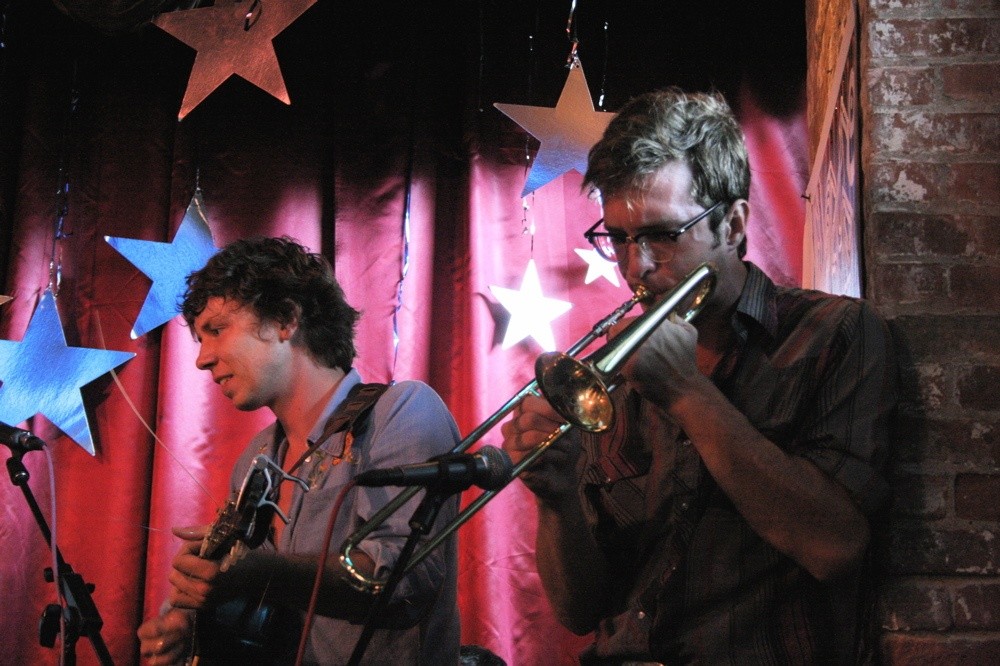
[500,91,897,666]
[138,233,465,666]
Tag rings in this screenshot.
[148,656,156,666]
[156,640,164,653]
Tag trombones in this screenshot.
[337,259,719,597]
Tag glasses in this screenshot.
[583,200,726,264]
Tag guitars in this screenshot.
[163,455,311,666]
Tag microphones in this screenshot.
[354,445,513,493]
[0,422,48,452]
